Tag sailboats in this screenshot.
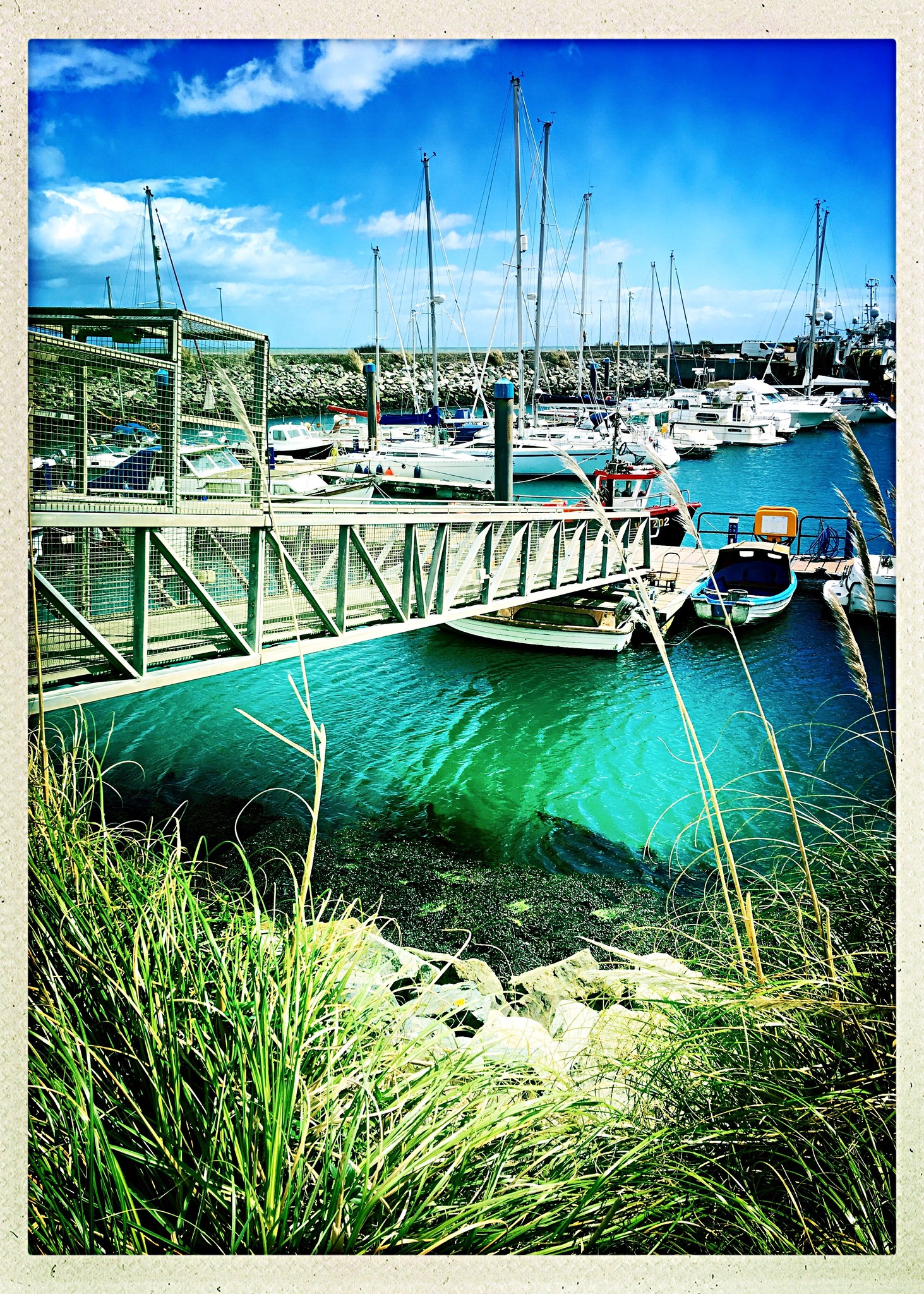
[33,70,897,543]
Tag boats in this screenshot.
[437,595,638,660]
[822,554,896,624]
[690,539,798,628]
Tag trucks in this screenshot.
[739,340,786,361]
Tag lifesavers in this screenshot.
[305,422,311,431]
[735,393,743,403]
[627,465,656,474]
[605,396,610,400]
